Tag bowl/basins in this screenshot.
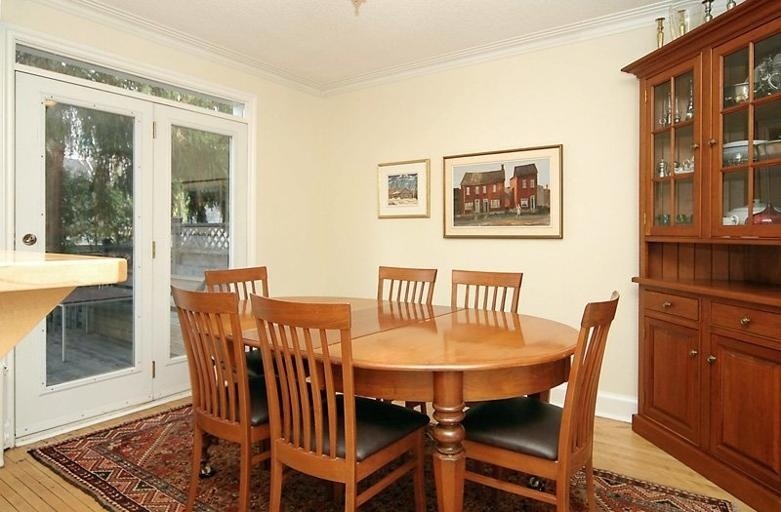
[722,80,750,106]
[722,138,781,166]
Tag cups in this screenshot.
[722,214,739,226]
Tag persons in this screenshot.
[515,204,521,219]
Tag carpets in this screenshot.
[25,404,733,512]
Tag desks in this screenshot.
[51,287,132,363]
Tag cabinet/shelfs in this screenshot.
[620,0,781,246]
[633,277,781,512]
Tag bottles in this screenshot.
[664,77,695,124]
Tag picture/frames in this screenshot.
[443,144,563,238]
[377,159,430,218]
